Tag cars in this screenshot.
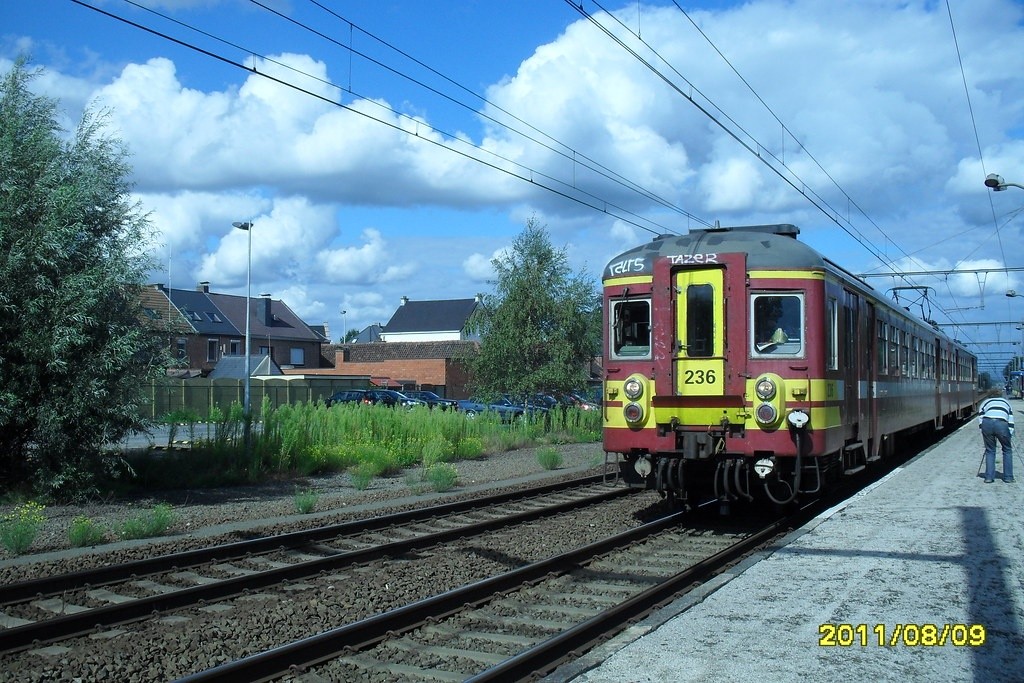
[314,388,400,408]
[456,393,552,424]
[520,391,600,414]
[380,389,429,410]
[402,390,460,412]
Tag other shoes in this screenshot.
[985,479,994,483]
[1003,479,1016,484]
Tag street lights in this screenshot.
[340,310,346,344]
[231,221,251,444]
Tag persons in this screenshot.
[1005,384,1011,395]
[979,397,1017,483]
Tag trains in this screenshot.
[600,222,979,518]
